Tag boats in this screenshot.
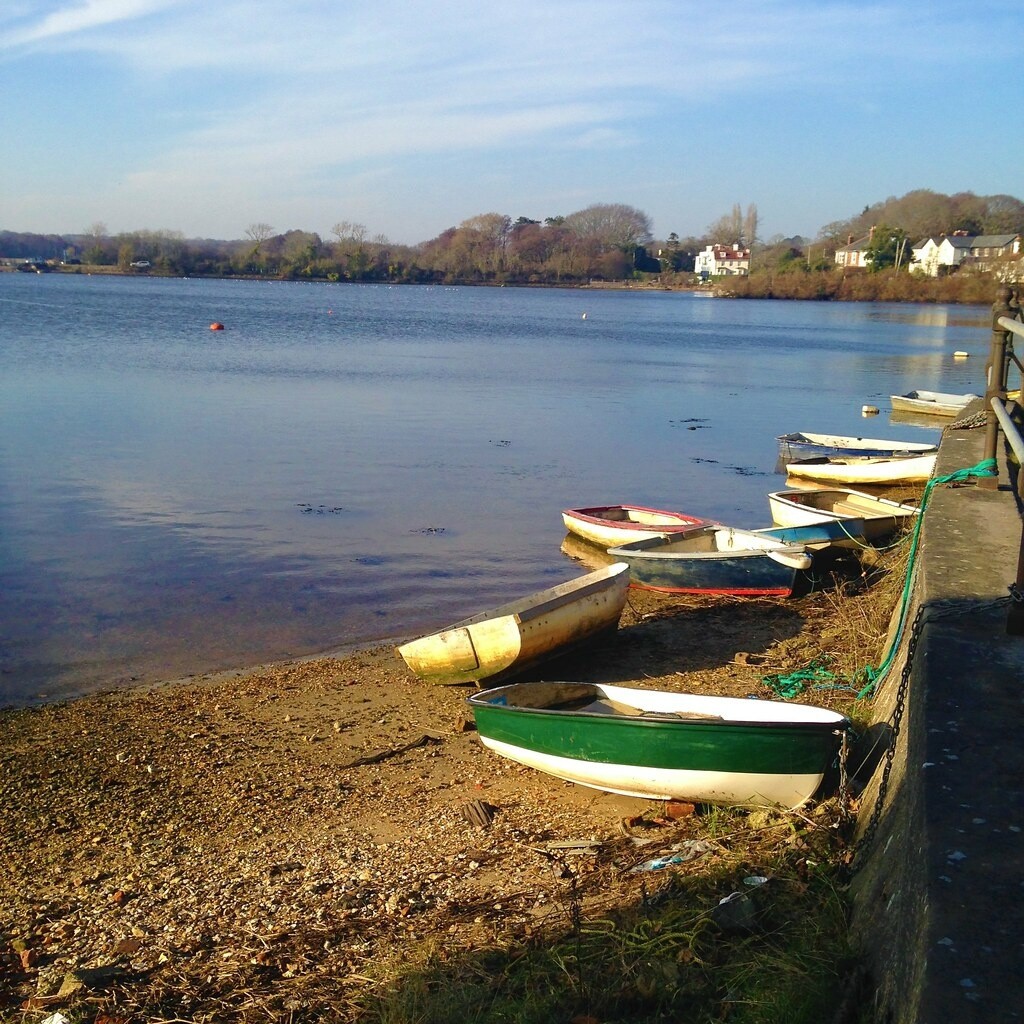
[891,389,1020,418]
[558,501,718,550]
[464,678,850,815]
[785,455,938,498]
[748,515,867,563]
[605,524,809,599]
[396,560,634,686]
[766,487,928,546]
[776,429,938,459]
